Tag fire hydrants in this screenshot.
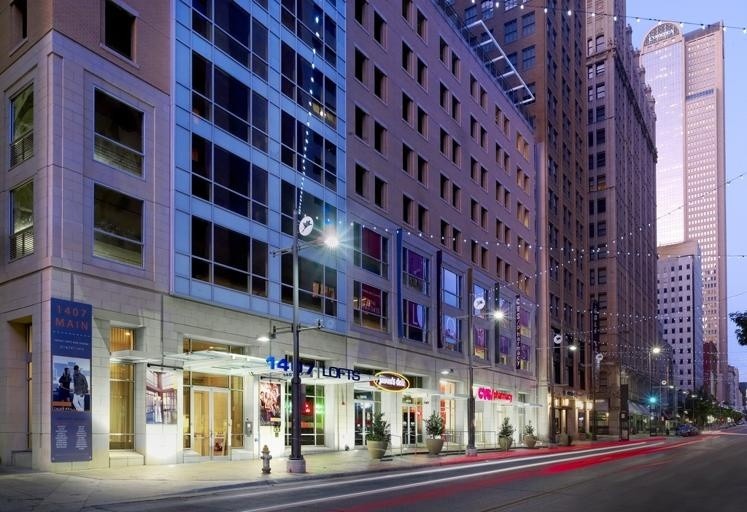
[259,443,273,474]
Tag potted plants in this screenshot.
[524,421,537,449]
[497,417,515,451]
[422,410,446,455]
[362,409,392,460]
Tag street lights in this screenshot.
[659,380,747,437]
[647,346,659,436]
[439,265,505,457]
[582,354,615,441]
[535,339,577,442]
[265,207,344,474]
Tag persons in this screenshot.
[73,366,88,412]
[59,368,72,402]
